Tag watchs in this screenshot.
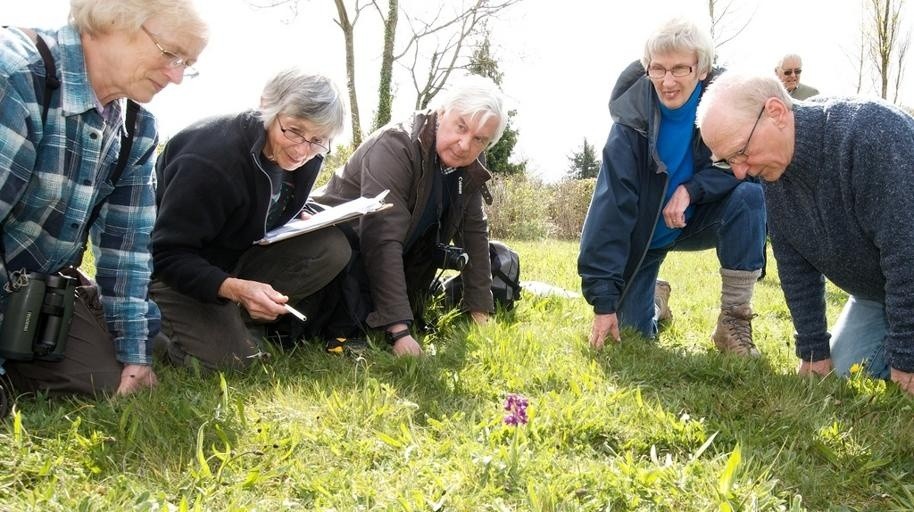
[385,328,410,346]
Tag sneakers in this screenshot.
[712,308,761,358]
[654,280,672,334]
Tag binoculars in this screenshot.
[0,271,77,363]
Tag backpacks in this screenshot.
[437,241,521,314]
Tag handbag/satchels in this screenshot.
[74,271,171,366]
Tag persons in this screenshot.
[578,19,768,364]
[307,74,507,364]
[149,67,352,384]
[695,67,913,396]
[756,53,820,281]
[0,0,209,408]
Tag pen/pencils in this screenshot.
[280,303,307,321]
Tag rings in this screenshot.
[672,219,676,223]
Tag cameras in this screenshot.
[434,245,470,271]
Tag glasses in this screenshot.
[645,62,697,77]
[711,110,764,169]
[275,117,332,154]
[141,27,199,81]
[781,67,802,77]
